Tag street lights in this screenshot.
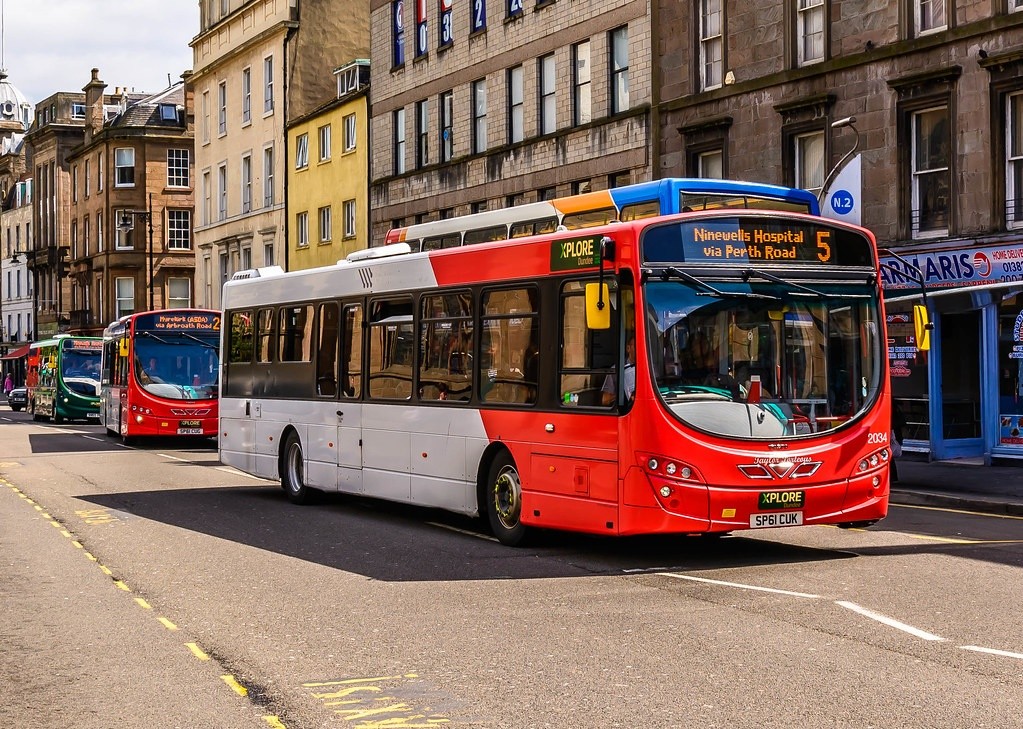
[9,237,39,342]
[120,192,155,311]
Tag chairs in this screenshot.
[357,364,529,406]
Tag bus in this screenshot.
[96,307,255,446]
[215,177,935,550]
[24,332,119,425]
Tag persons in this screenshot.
[141,356,163,384]
[65,359,83,377]
[4,373,15,397]
[600,332,636,406]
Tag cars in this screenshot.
[7,385,29,412]
